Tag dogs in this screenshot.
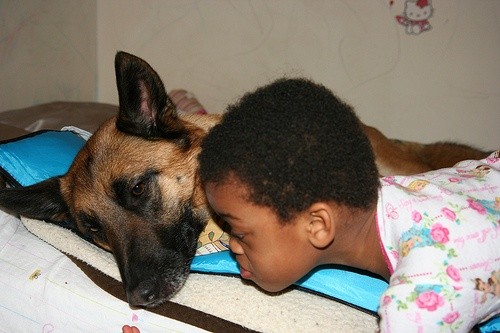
[0,51,497,310]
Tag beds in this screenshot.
[0,96,500,333]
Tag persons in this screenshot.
[121,77,500,333]
